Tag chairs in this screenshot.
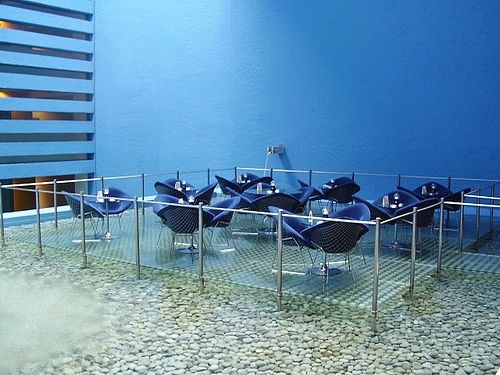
[249,193,304,237]
[193,182,218,206]
[164,178,196,197]
[286,185,314,209]
[154,181,190,202]
[413,181,455,227]
[398,184,435,230]
[231,172,260,187]
[325,202,371,272]
[433,187,472,231]
[241,183,271,202]
[392,197,440,254]
[372,190,421,248]
[225,186,262,235]
[83,186,133,230]
[321,177,355,195]
[365,199,397,249]
[298,216,370,287]
[297,180,326,215]
[61,191,105,239]
[240,176,273,194]
[152,194,192,250]
[202,195,242,248]
[156,204,215,265]
[325,183,361,214]
[215,174,242,194]
[267,205,315,274]
